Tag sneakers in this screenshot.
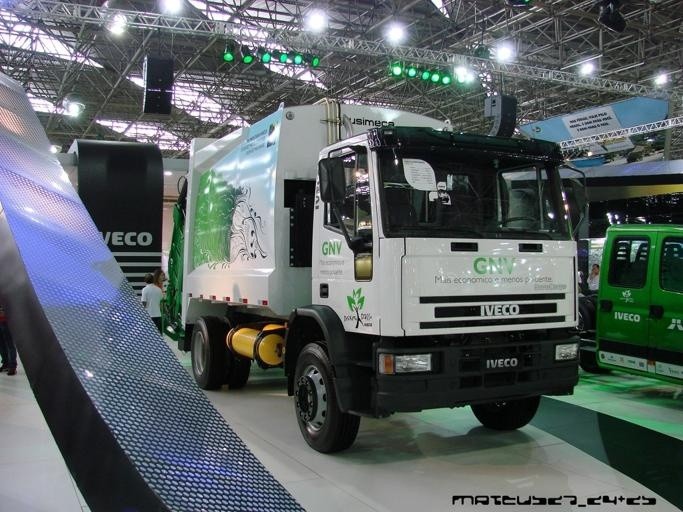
[0,365,17,375]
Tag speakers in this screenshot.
[140,55,174,116]
[482,92,520,140]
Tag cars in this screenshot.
[630,132,665,153]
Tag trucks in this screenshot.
[579,223,683,390]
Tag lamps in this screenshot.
[221,40,321,69]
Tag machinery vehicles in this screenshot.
[149,93,591,457]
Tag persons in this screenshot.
[586,263,600,291]
[140,273,170,338]
[577,268,584,286]
[153,270,166,296]
[0,304,17,375]
